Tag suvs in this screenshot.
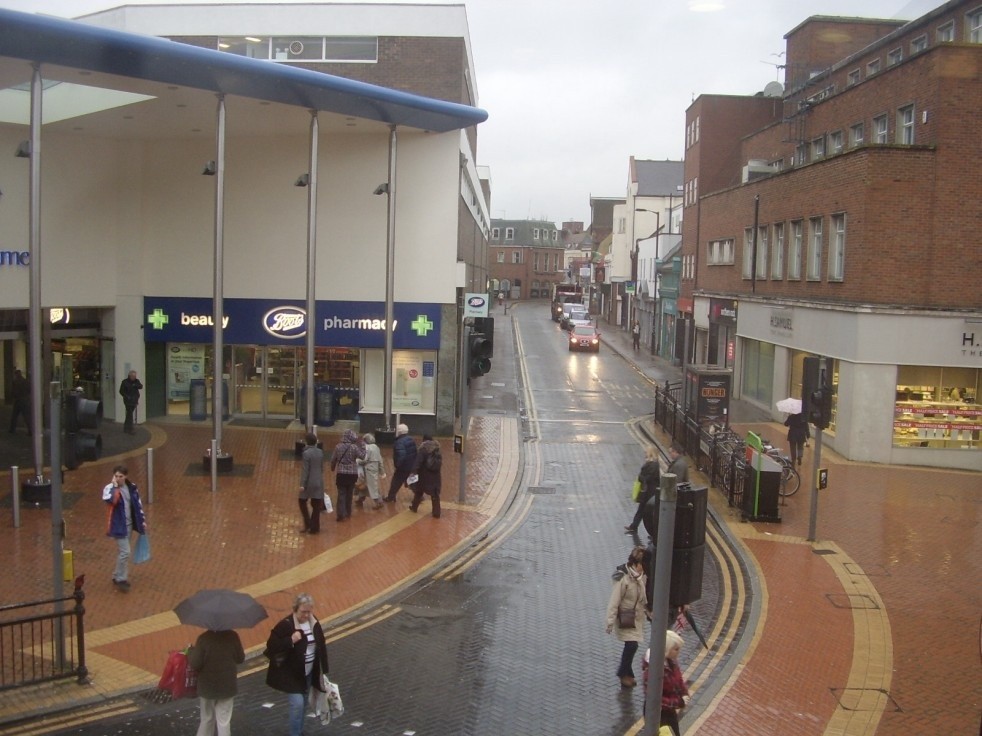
[558,302,593,331]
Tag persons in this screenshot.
[498,292,504,304]
[632,320,641,350]
[294,423,442,535]
[784,413,811,466]
[184,628,246,736]
[119,370,143,435]
[102,465,147,594]
[606,441,692,736]
[263,592,329,735]
[8,370,34,437]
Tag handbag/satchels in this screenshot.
[308,677,332,725]
[618,609,636,628]
[355,481,366,490]
[158,647,189,691]
[172,648,199,700]
[632,476,641,503]
[133,532,150,564]
[323,674,344,720]
[263,619,293,667]
[322,492,335,514]
[806,438,810,448]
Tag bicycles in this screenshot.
[690,414,801,497]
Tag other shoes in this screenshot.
[625,526,636,532]
[373,503,384,510]
[300,524,310,533]
[797,457,801,465]
[620,675,637,687]
[112,578,130,587]
[115,581,129,593]
[384,497,396,502]
[337,515,351,522]
[409,505,418,513]
[355,500,363,505]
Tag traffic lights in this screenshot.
[59,389,105,470]
[809,388,831,429]
[467,332,493,377]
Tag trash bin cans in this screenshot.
[744,446,782,516]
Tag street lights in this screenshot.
[635,208,659,355]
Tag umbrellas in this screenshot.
[173,588,269,633]
[681,604,711,658]
[775,398,803,415]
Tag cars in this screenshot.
[567,325,602,353]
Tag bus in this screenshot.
[550,282,588,322]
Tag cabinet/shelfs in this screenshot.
[280,357,352,380]
[896,402,982,443]
